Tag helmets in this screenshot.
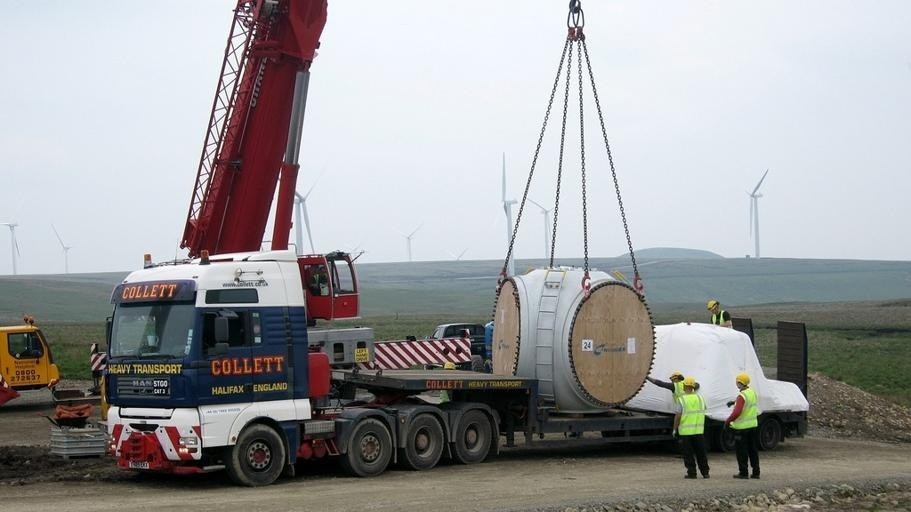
[707,301,719,311]
[669,371,699,389]
[736,373,750,385]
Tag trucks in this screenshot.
[101,243,809,486]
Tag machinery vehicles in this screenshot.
[1,315,61,406]
[88,0,376,401]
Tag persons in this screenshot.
[724,374,760,479]
[647,373,700,457]
[673,377,710,479]
[707,300,732,329]
[309,268,327,296]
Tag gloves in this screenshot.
[727,401,735,407]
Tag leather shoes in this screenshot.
[733,473,760,478]
[685,474,710,478]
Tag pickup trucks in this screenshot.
[422,317,486,372]
